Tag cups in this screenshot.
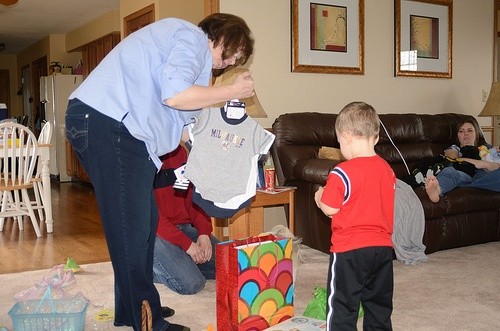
[265,169,275,191]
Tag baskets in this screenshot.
[8,283,89,331]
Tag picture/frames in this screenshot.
[393,0,454,79]
[290,0,367,75]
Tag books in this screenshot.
[257,185,298,195]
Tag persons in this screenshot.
[425,116,500,202]
[153,144,221,295]
[314,102,397,331]
[66,14,256,331]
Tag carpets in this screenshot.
[0,235,500,330]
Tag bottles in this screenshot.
[426,166,432,178]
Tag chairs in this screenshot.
[0,119,54,237]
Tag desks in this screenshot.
[208,185,296,245]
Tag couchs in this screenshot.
[271,111,500,261]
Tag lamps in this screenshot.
[209,66,267,119]
[477,82,500,118]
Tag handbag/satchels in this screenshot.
[215,231,295,331]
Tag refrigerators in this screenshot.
[40,73,83,183]
